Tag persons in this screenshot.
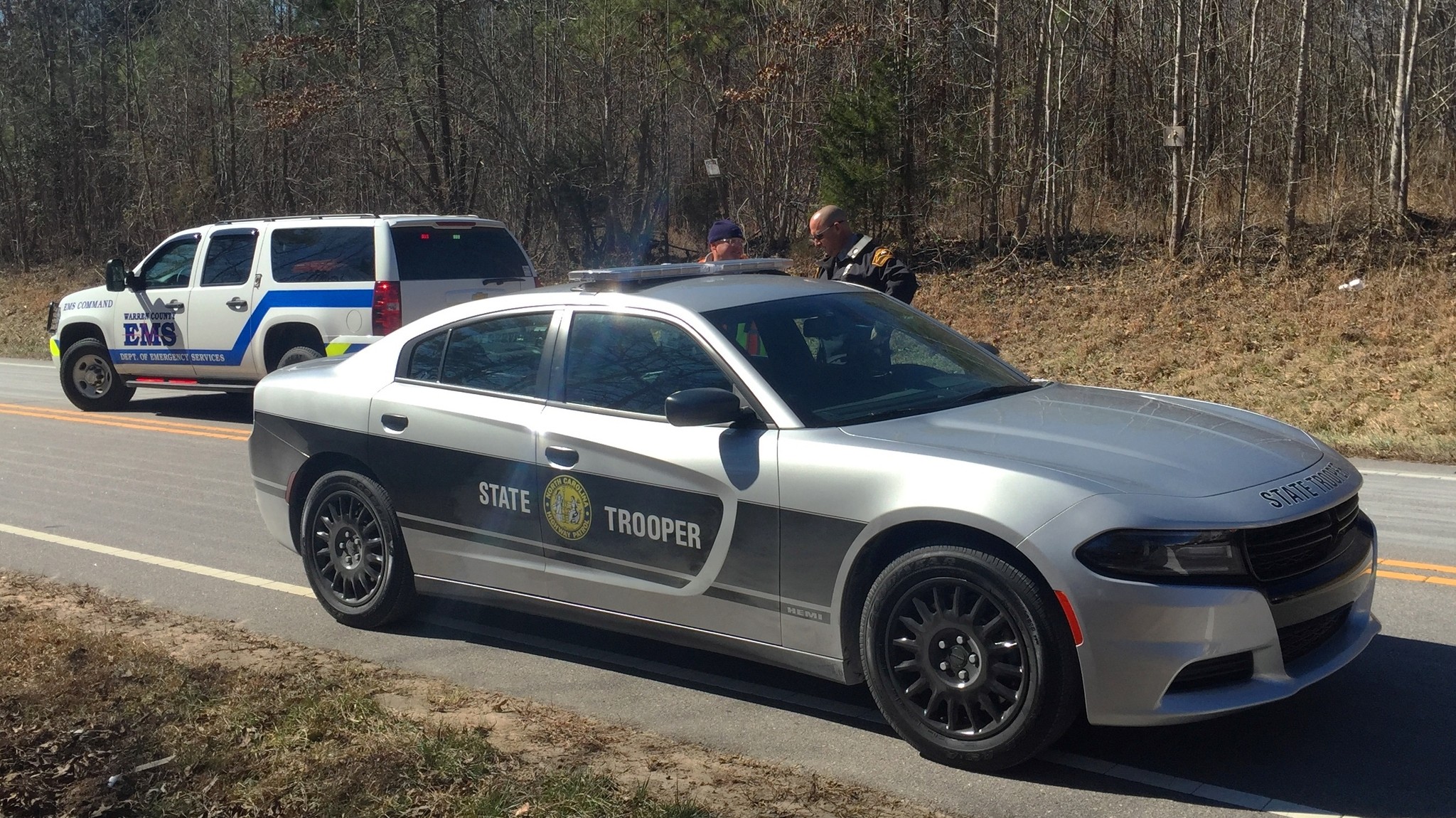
[697,220,749,341]
[803,205,918,364]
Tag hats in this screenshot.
[707,219,746,244]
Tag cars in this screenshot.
[245,256,1384,774]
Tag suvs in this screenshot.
[44,211,546,412]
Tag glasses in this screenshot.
[810,220,846,241]
[720,238,747,247]
